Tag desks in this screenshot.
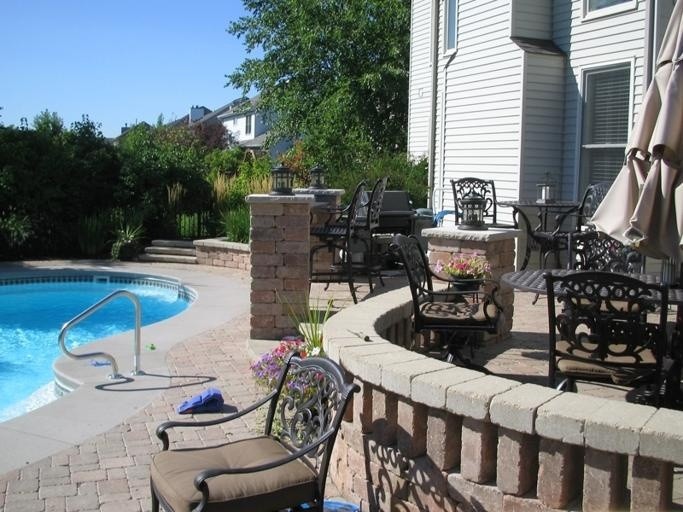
[500,268,683,345]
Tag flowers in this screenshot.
[433,249,495,280]
[249,283,330,448]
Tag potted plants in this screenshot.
[107,220,147,263]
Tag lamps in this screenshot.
[309,162,328,189]
[270,160,295,196]
[458,192,488,231]
[536,182,557,205]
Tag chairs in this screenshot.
[542,270,669,409]
[148,350,361,512]
[449,177,516,228]
[389,233,504,368]
[308,179,368,305]
[567,178,647,276]
[321,178,386,294]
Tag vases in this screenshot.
[451,279,481,296]
[296,397,330,432]
